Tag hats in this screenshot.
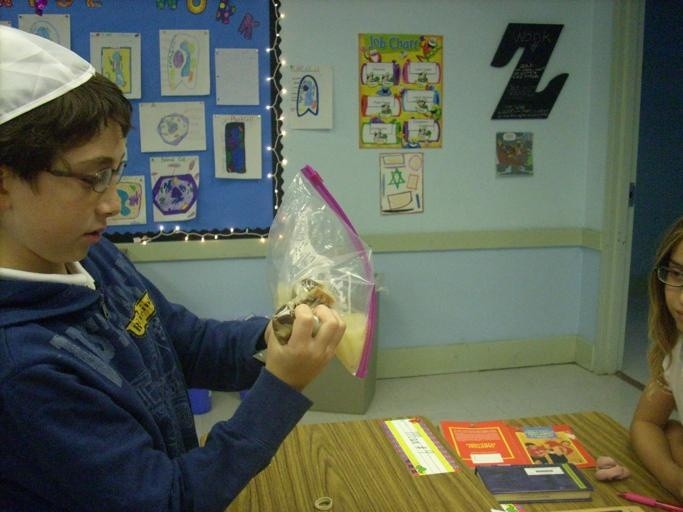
[0,24,95,126]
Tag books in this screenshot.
[439,418,596,504]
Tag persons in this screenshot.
[0,22,347,512]
[628,215,683,505]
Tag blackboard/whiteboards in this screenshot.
[0,3,599,266]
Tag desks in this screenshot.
[194,413,503,510]
[436,411,682,510]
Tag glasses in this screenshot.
[42,158,130,194]
[653,266,683,288]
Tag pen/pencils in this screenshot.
[616,491,682,512]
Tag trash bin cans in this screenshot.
[273,277,377,415]
